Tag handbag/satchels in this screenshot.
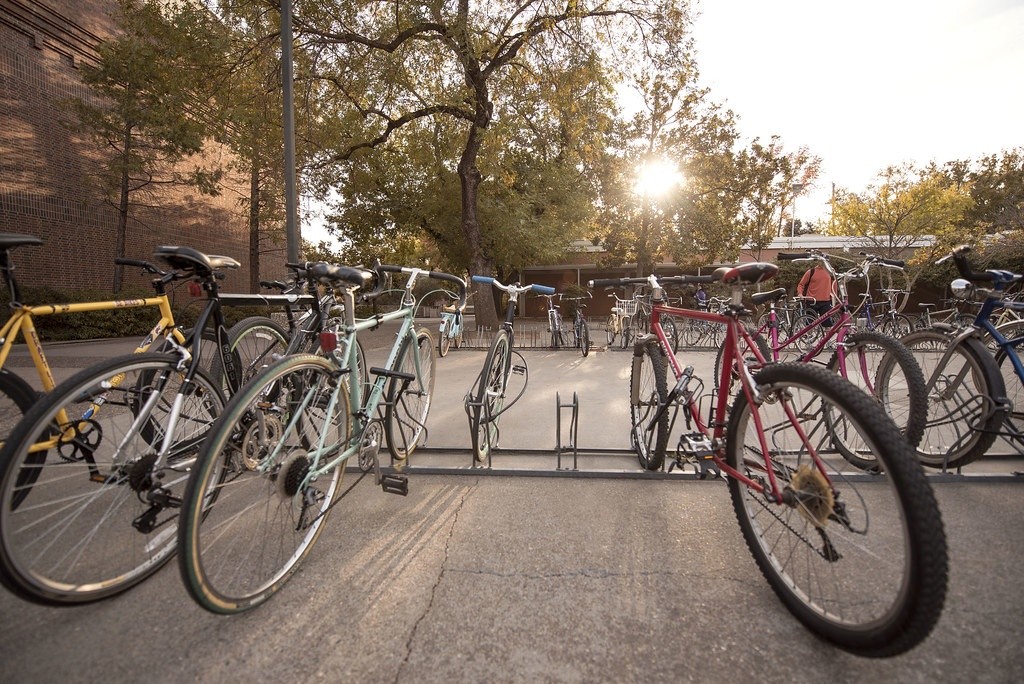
[794,268,815,302]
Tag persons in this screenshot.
[797,254,838,349]
[693,284,707,311]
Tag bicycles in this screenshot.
[559,291,594,357]
[1,231,244,514]
[588,261,951,660]
[589,243,1024,477]
[534,292,567,348]
[438,287,471,358]
[176,258,471,615]
[461,275,556,470]
[0,243,395,607]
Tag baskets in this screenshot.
[615,300,637,316]
